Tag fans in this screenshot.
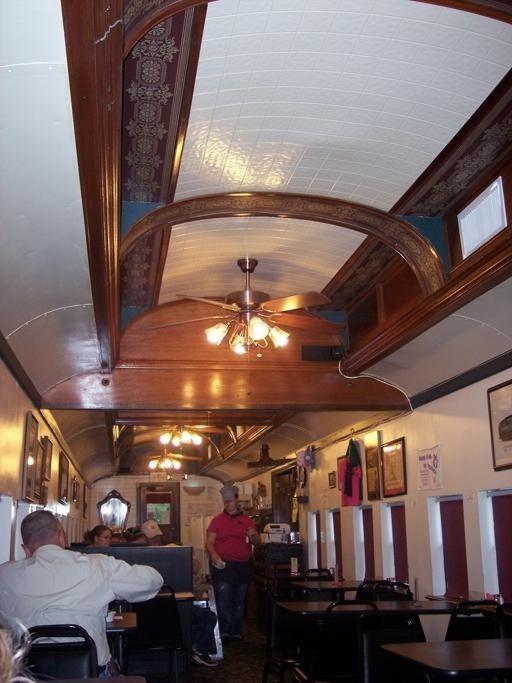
[133,424,225,480]
[146,258,346,335]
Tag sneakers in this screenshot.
[191,653,219,667]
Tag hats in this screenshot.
[220,486,238,500]
[141,519,163,539]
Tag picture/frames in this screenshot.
[22,410,53,507]
[380,436,407,498]
[364,446,380,501]
[328,471,336,489]
[487,378,512,471]
[57,451,88,519]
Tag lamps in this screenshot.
[205,311,291,358]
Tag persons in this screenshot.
[84,524,112,547]
[141,520,216,668]
[123,526,147,544]
[0,509,165,677]
[205,483,261,641]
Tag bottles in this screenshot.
[499,592,505,606]
[329,568,334,575]
[281,528,288,544]
[392,577,398,590]
[244,527,255,543]
[494,594,500,605]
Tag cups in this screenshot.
[114,603,126,616]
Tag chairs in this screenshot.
[261,567,511,682]
[17,542,195,683]
